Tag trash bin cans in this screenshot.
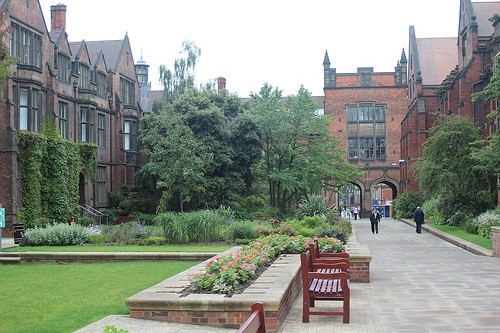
[13,223,25,244]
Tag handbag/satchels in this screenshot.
[421,218,425,224]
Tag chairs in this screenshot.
[237,302,267,333]
[301,239,352,323]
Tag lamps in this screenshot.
[392,163,401,167]
[399,160,407,163]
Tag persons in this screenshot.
[339,206,343,213]
[370,207,380,234]
[350,203,361,220]
[413,206,424,233]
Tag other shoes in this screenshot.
[376,231,378,234]
[416,231,421,234]
